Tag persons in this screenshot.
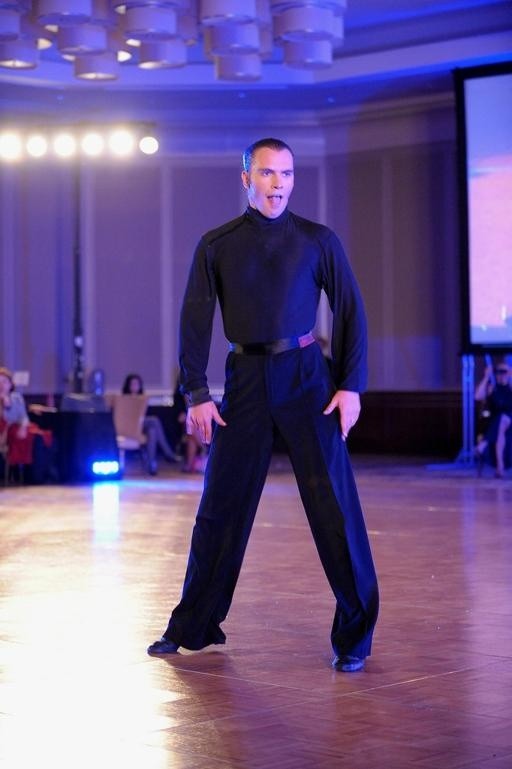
[146,138,379,673]
[0,368,53,485]
[471,362,511,479]
[172,369,209,473]
[120,374,184,476]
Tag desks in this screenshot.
[26,412,121,480]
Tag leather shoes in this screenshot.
[148,636,179,654]
[331,654,364,672]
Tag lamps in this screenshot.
[0,0,350,83]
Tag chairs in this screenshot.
[112,395,149,472]
